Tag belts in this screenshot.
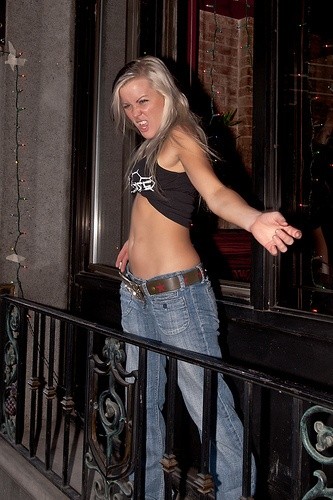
[117,263,206,295]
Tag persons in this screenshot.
[307,95,333,289]
[109,55,302,500]
